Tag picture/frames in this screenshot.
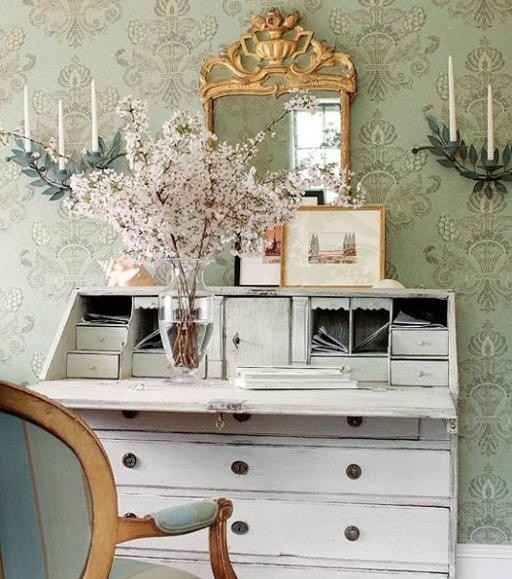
[233,190,388,288]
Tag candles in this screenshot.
[447,54,457,141]
[57,99,66,169]
[90,79,99,151]
[23,84,32,152]
[486,83,494,161]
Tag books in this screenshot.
[309,326,348,355]
[352,321,390,352]
[228,363,360,392]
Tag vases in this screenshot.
[156,258,217,385]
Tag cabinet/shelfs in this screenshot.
[21,287,461,579]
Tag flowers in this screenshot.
[0,90,374,368]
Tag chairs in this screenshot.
[0,380,238,579]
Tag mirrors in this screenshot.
[194,6,358,230]
